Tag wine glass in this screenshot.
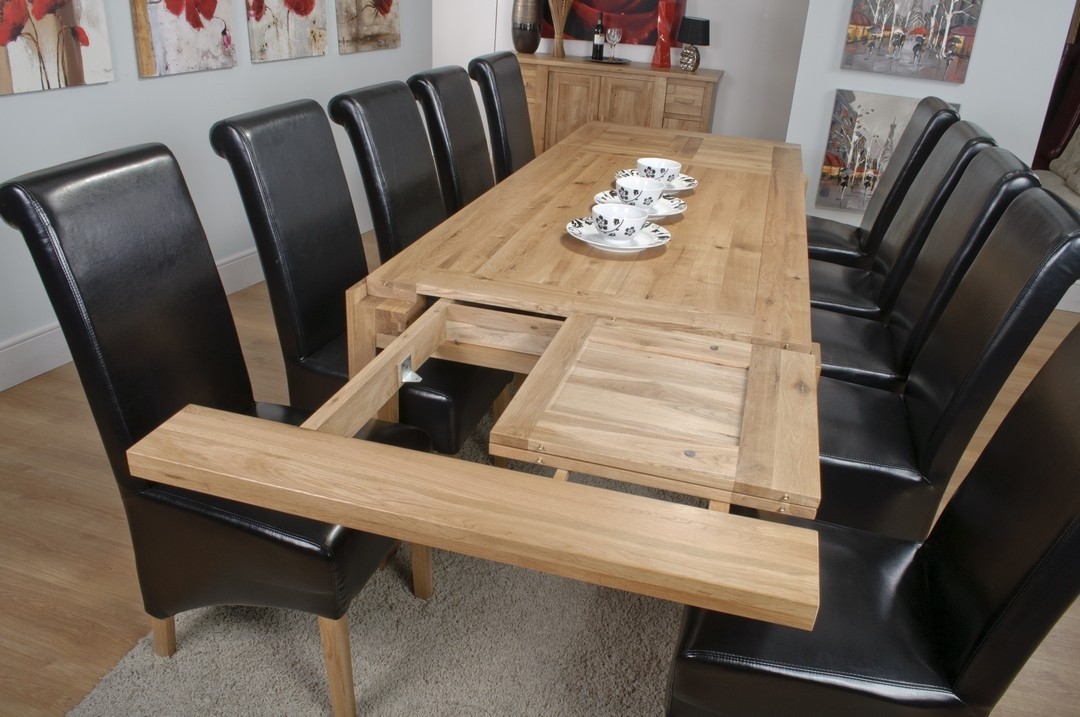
[606,28,622,62]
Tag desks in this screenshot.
[121,119,828,636]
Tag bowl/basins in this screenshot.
[637,157,682,186]
[591,203,648,243]
[616,176,665,210]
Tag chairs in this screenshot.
[0,49,540,717]
[664,95,1080,717]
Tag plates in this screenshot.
[583,55,631,63]
[594,189,689,220]
[615,169,700,195]
[566,216,673,253]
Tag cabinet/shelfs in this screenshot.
[513,51,725,162]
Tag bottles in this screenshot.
[591,10,605,60]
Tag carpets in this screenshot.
[53,406,711,717]
